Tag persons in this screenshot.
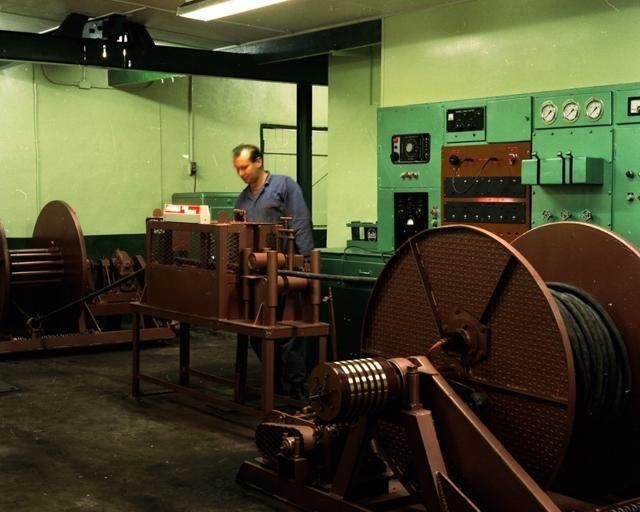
[232,142,317,404]
